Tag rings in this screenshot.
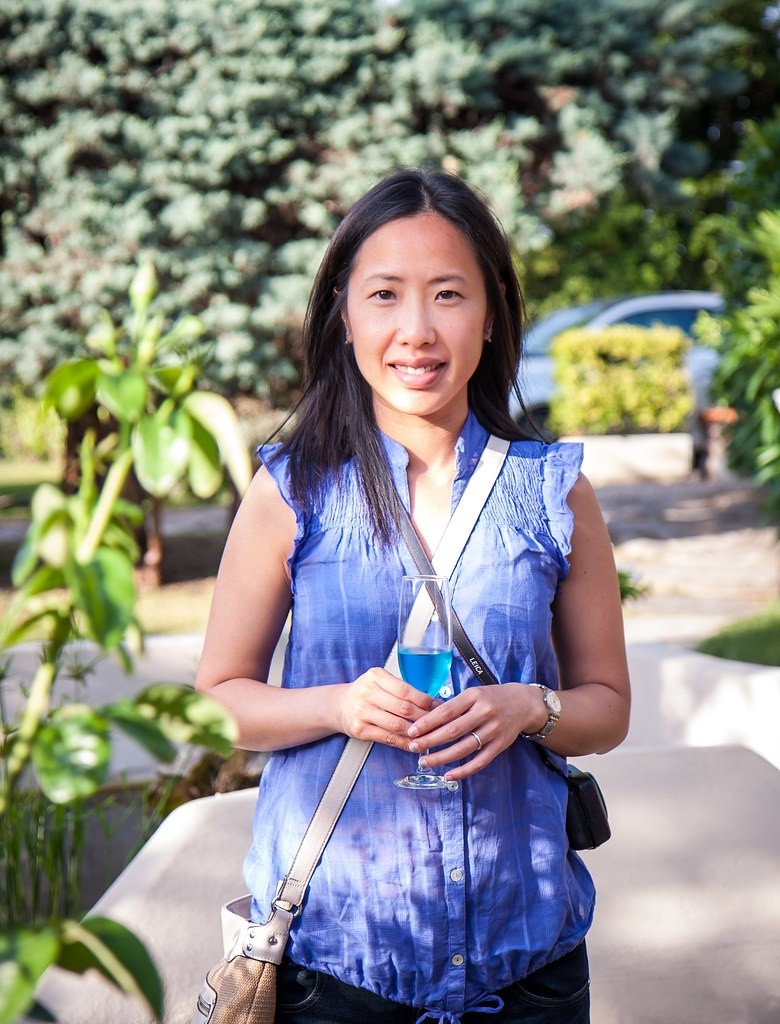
[470,731,483,750]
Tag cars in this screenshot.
[506,287,729,440]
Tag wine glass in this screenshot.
[394,574,457,789]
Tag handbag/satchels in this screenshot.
[182,898,283,1024]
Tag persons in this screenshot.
[192,170,633,1024]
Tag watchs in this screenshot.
[516,680,563,742]
